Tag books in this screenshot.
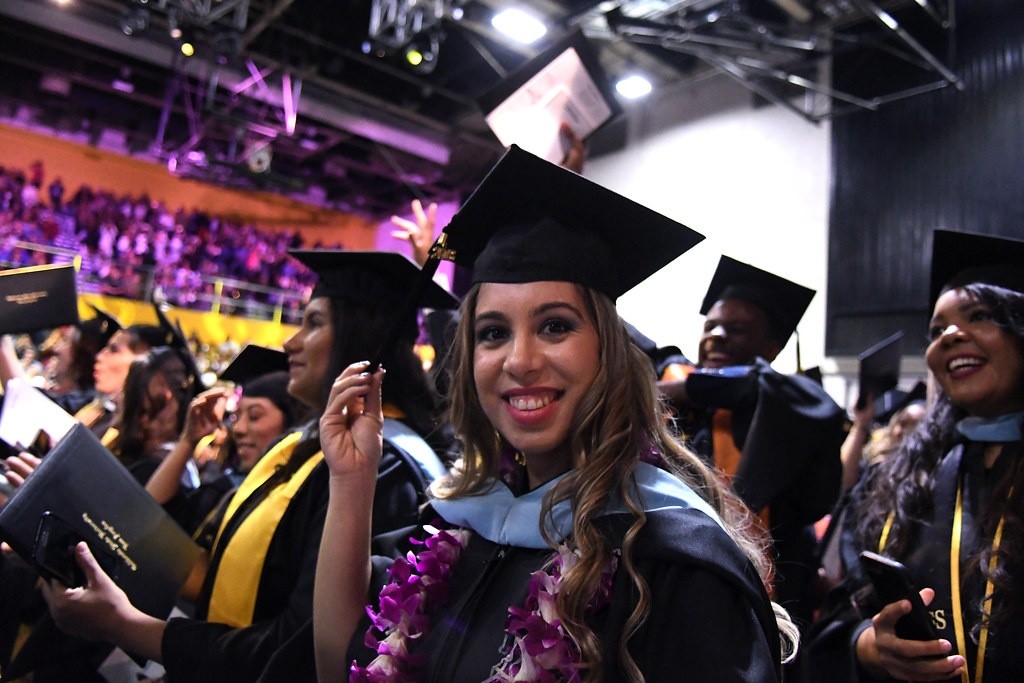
[1,377,78,455]
[1,421,201,669]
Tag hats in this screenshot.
[219,344,300,408]
[872,380,928,427]
[368,144,706,377]
[930,228,1024,317]
[76,300,209,409]
[699,254,816,353]
[289,249,461,340]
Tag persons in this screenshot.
[1,145,1024,683]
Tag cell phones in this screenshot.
[859,551,948,663]
[32,512,118,589]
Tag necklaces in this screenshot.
[352,427,658,683]
